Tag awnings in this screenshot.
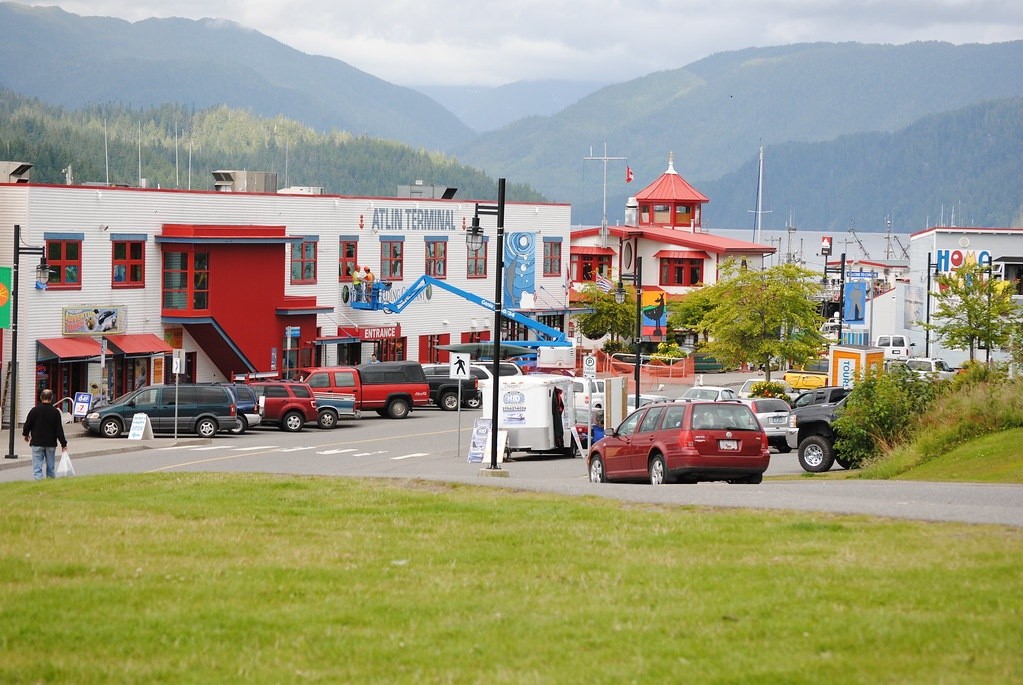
[105,333,174,358]
[35,337,114,364]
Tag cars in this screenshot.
[624,316,962,472]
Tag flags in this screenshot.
[566,264,574,293]
[595,272,614,294]
[843,282,865,325]
[0,267,13,328]
[903,284,925,332]
[641,291,666,343]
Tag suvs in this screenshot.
[583,399,774,487]
[415,349,577,410]
[233,379,318,432]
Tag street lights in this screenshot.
[467,176,510,471]
[925,251,942,357]
[822,252,847,345]
[3,224,49,460]
[613,255,644,410]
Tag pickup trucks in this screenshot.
[310,391,357,428]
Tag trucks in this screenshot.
[301,362,430,419]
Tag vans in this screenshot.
[80,382,238,438]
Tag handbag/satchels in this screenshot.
[54,448,75,477]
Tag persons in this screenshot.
[22,389,68,480]
[351,265,364,301]
[367,354,380,363]
[362,266,376,303]
